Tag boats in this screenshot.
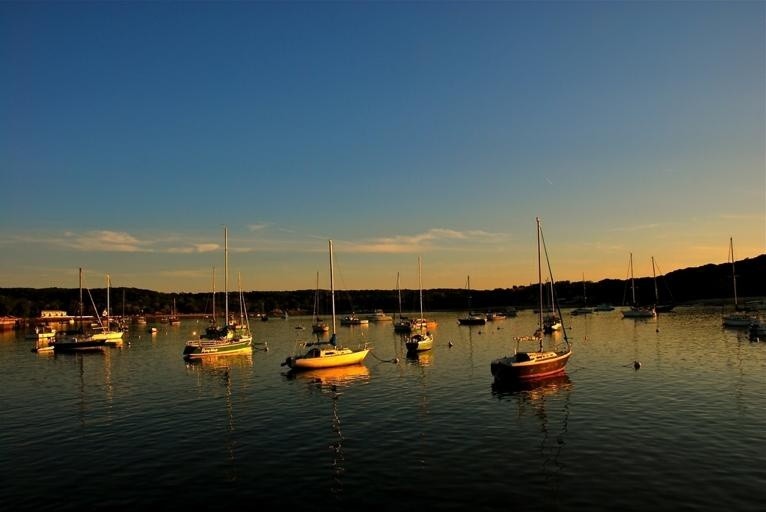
[130,310,147,324]
[365,309,393,322]
[744,300,765,312]
[485,312,506,322]
[503,307,520,318]
[750,313,766,337]
[592,303,615,311]
[416,318,438,328]
[340,312,370,324]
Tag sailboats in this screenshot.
[721,233,755,329]
[286,365,372,389]
[160,298,181,326]
[404,255,434,352]
[491,215,573,387]
[569,272,594,315]
[279,239,373,372]
[649,257,674,313]
[310,270,331,334]
[182,227,257,363]
[533,284,557,314]
[457,275,488,325]
[619,253,657,318]
[393,271,418,334]
[22,265,128,354]
